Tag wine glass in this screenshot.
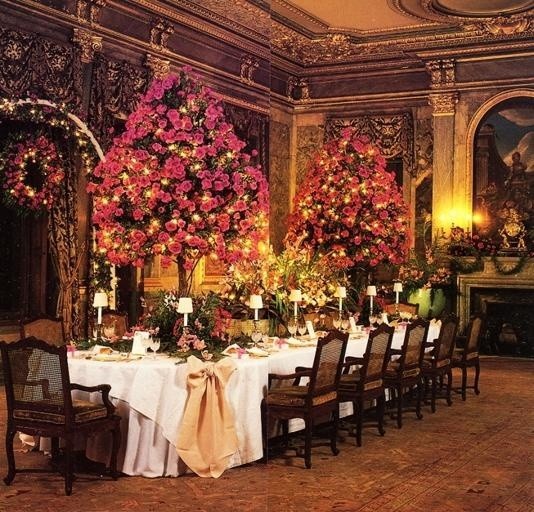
[249,316,349,350]
[366,311,412,327]
[140,332,161,360]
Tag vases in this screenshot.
[407,288,446,319]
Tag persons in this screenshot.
[505,152,527,189]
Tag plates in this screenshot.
[94,356,122,361]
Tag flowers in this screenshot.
[387,260,451,289]
[283,124,418,268]
[3,134,68,213]
[132,291,232,362]
[425,233,486,260]
[87,65,269,273]
[217,248,342,310]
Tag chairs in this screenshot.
[0,335,121,496]
[258,315,486,477]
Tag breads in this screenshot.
[227,347,239,354]
[100,348,113,355]
